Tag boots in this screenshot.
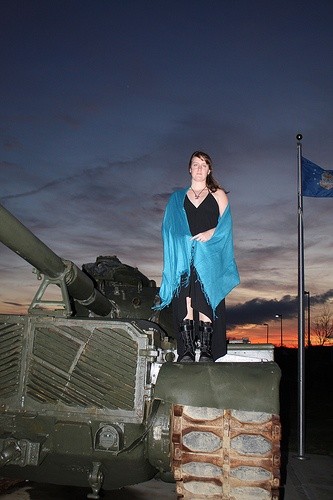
[178,319,197,362]
[198,320,214,362]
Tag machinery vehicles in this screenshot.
[1,204,286,500]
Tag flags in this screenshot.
[300,156,333,198]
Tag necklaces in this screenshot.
[189,185,208,200]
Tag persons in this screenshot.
[160,150,241,362]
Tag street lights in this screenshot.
[264,323,269,344]
[304,290,311,347]
[275,314,284,346]
